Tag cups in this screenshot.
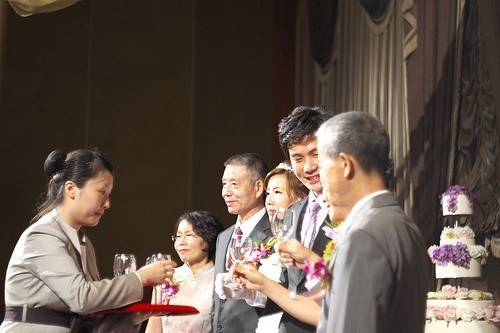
[113,253,136,277]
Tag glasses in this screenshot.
[171,233,198,241]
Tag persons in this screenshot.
[215,106,342,333]
[144,210,226,333]
[273,111,431,333]
[228,154,396,333]
[257,158,311,333]
[0,147,177,333]
[210,151,277,333]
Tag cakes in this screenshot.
[424,185,500,333]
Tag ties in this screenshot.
[302,202,322,249]
[227,227,243,269]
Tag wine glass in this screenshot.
[145,253,172,305]
[224,236,252,288]
[270,210,294,266]
